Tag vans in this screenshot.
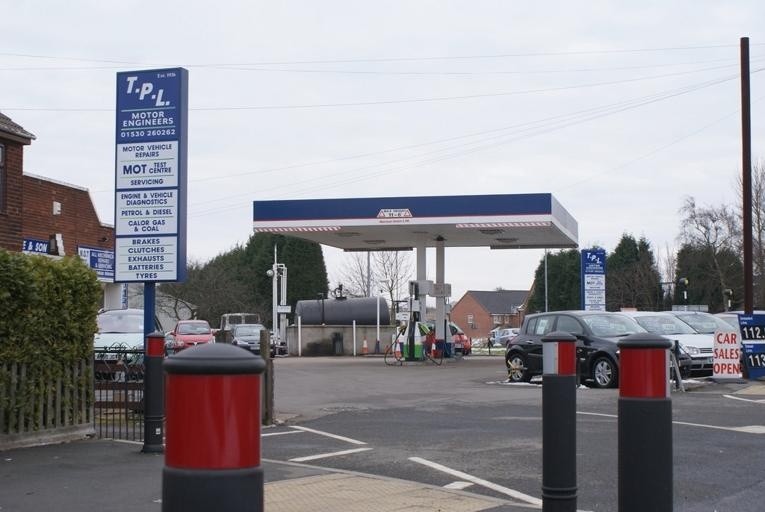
[397,322,471,355]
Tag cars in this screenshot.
[170,319,219,355]
[500,310,765,388]
[232,324,276,358]
[93,308,168,380]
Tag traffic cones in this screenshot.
[395,340,402,358]
[362,336,368,353]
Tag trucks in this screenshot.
[220,313,260,331]
